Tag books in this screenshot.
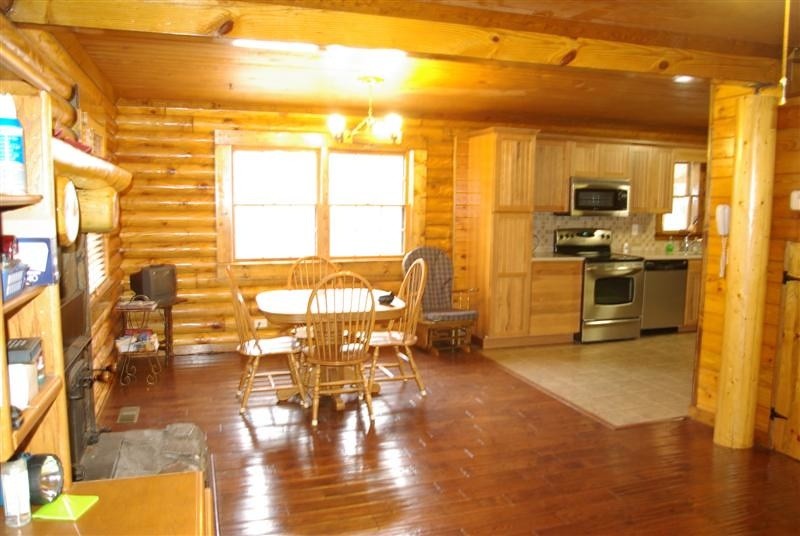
[115,300,158,311]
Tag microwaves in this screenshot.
[554,176,632,218]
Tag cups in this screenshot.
[0,457,31,528]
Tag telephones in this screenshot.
[715,205,729,235]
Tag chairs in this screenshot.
[225,247,479,427]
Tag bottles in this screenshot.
[666,236,673,254]
[623,240,628,253]
[1,118,28,196]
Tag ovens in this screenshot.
[576,261,644,344]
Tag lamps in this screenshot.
[327,76,404,148]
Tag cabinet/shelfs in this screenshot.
[467,127,674,350]
[0,14,216,536]
[677,259,702,333]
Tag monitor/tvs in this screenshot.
[130,265,177,303]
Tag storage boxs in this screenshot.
[1,263,30,302]
[6,338,42,365]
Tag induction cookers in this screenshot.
[553,228,643,263]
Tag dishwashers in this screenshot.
[640,260,688,334]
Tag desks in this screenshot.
[123,297,190,369]
[256,287,406,401]
